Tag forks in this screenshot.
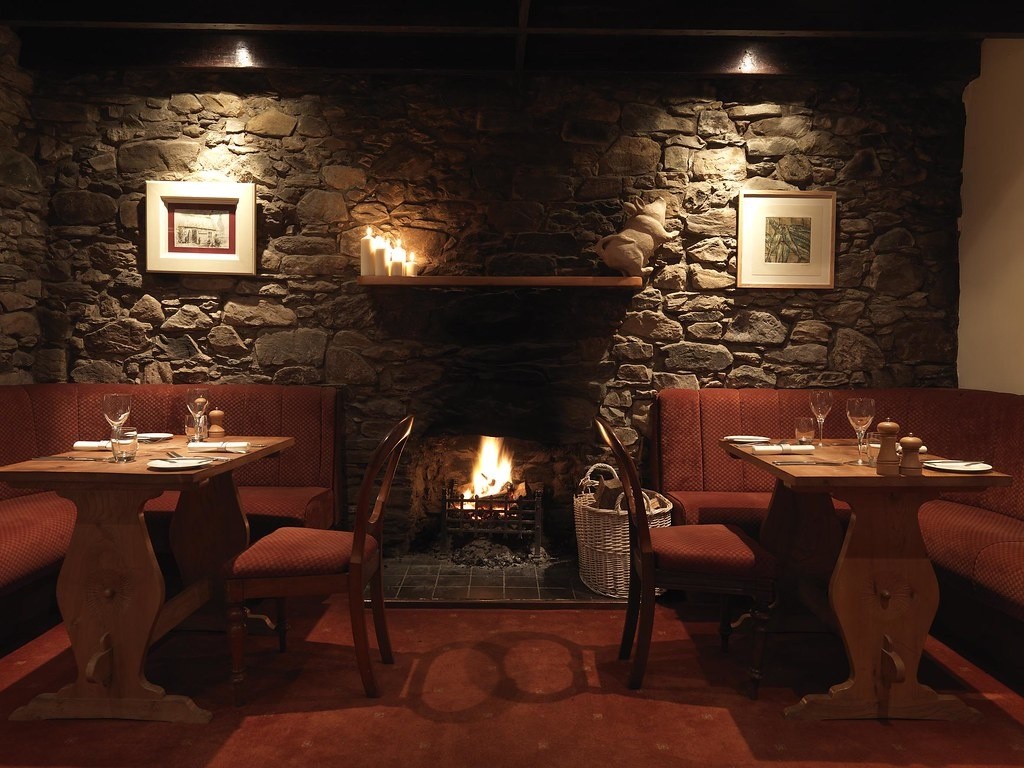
[137,437,165,444]
[736,439,787,445]
[167,451,235,460]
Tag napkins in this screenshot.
[73,440,141,451]
[751,444,815,454]
[861,444,927,454]
[188,441,251,453]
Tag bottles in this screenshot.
[876,417,922,476]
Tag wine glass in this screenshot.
[103,393,131,438]
[808,391,832,447]
[843,398,875,466]
[186,388,210,439]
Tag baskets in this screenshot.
[573,463,673,599]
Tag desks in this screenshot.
[718,438,1013,725]
[0,434,293,725]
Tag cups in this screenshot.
[866,432,881,467]
[111,427,137,461]
[185,414,208,443]
[794,417,814,444]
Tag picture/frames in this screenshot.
[738,189,836,289]
[146,180,257,275]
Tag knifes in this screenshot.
[150,458,218,460]
[920,461,984,463]
[775,463,841,465]
[30,457,95,461]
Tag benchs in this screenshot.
[656,388,1024,694]
[0,381,344,659]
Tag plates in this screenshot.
[725,435,771,441]
[147,457,213,468]
[137,433,174,438]
[923,460,992,471]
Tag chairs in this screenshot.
[221,416,416,708]
[595,418,769,694]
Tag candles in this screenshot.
[361,234,418,276]
